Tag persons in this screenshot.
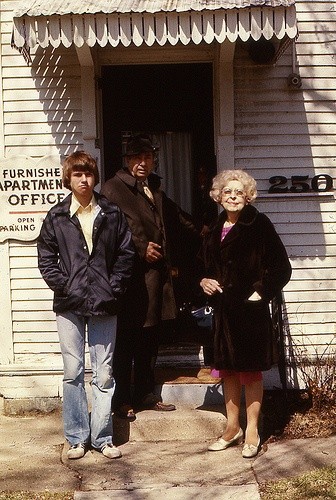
[185,168,293,459]
[100,139,211,421]
[37,151,134,460]
[140,165,218,369]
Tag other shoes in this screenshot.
[99,443,122,459]
[67,443,85,459]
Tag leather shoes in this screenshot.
[143,394,175,411]
[114,402,135,418]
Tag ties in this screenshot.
[136,179,156,207]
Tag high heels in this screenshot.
[242,434,260,458]
[208,427,243,451]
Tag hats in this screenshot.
[121,134,159,158]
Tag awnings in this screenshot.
[10,0,301,70]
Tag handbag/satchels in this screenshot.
[173,290,215,346]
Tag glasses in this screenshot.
[222,188,245,197]
